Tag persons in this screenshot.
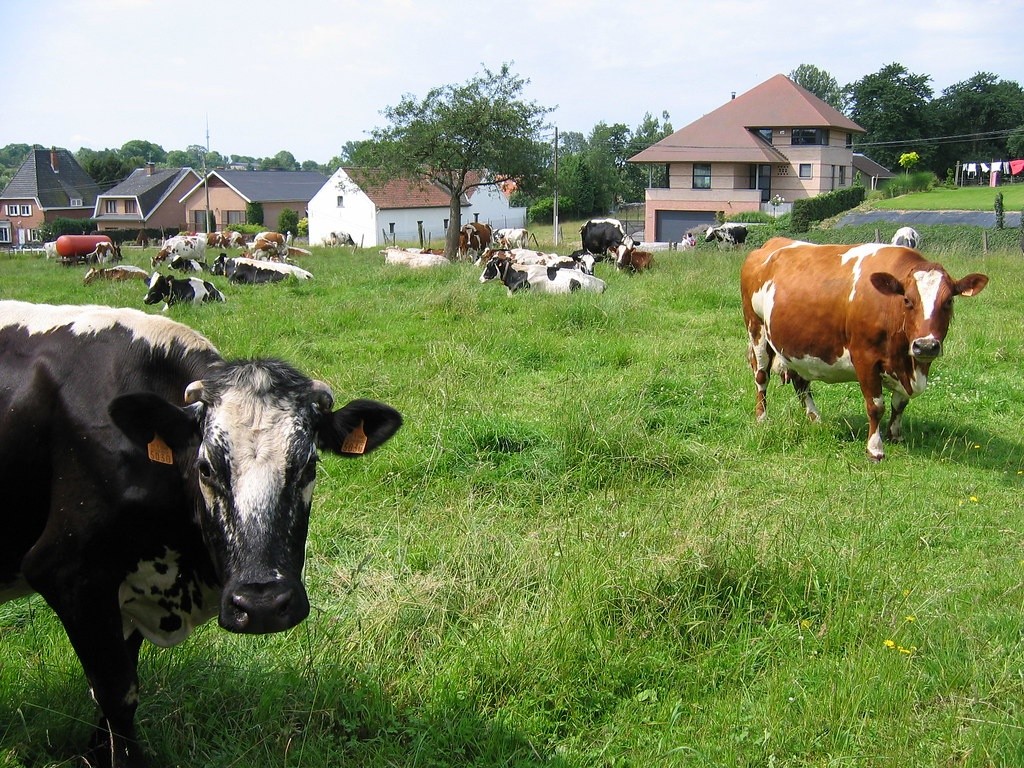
[681,235,686,251]
[286,230,293,245]
[686,232,695,247]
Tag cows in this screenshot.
[379,245,459,269]
[322,231,355,247]
[890,227,920,251]
[702,226,749,250]
[0,300,404,768]
[459,218,653,298]
[44,230,314,310]
[740,237,989,461]
